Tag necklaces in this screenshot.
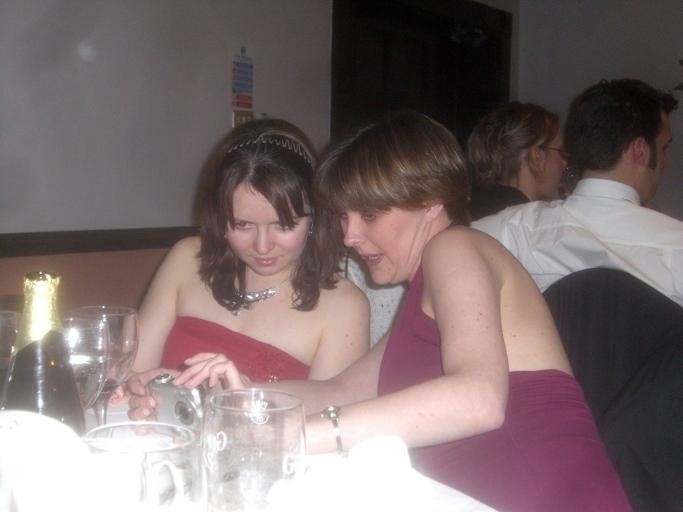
[226,280,289,314]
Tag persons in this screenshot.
[468,76,683,302]
[463,100,571,222]
[126,110,636,512]
[106,118,371,410]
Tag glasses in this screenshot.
[538,144,572,159]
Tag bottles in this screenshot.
[0,271,85,435]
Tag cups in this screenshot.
[96,420,196,498]
[202,387,309,492]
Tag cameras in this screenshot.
[145,373,206,442]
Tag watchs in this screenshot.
[321,403,348,455]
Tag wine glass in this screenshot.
[0,304,139,428]
